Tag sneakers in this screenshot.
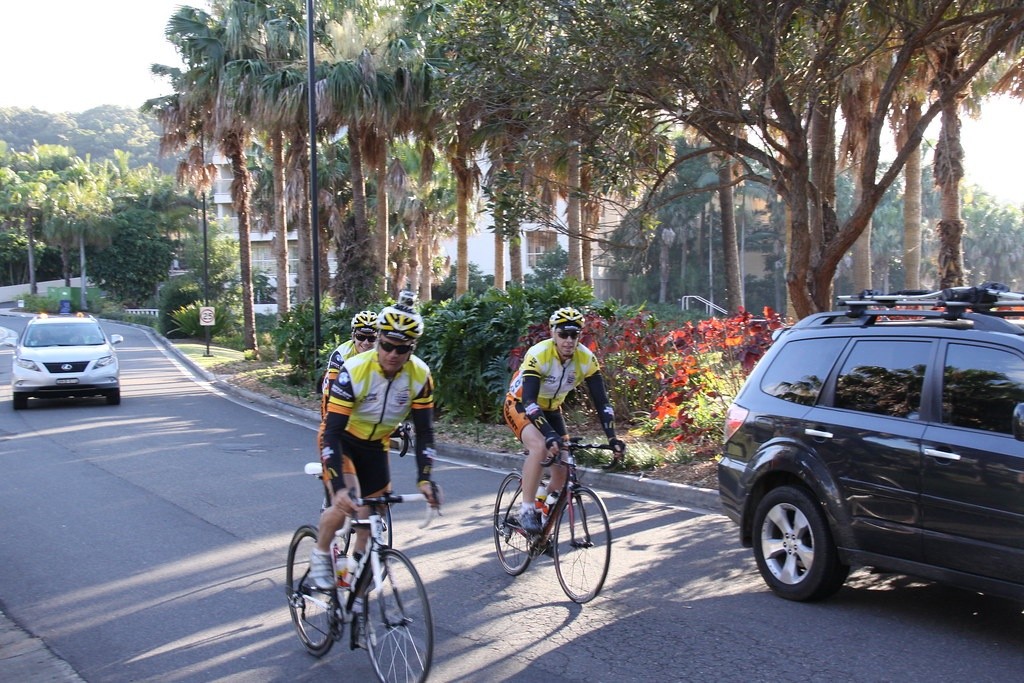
[542,534,559,557]
[355,615,378,649]
[516,507,544,532]
[307,548,335,590]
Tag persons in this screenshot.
[310,304,443,648]
[503,307,626,541]
[322,311,378,590]
[28,330,53,346]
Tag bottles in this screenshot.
[336,548,348,586]
[535,479,549,512]
[342,551,363,585]
[541,489,561,517]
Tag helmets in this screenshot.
[377,304,424,341]
[350,311,378,334]
[399,290,418,308]
[548,306,586,331]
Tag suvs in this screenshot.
[716,287,1024,604]
[4,312,124,409]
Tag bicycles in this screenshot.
[372,420,414,584]
[493,435,625,604]
[284,472,441,683]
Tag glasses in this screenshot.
[354,333,378,343]
[378,337,416,355]
[555,330,581,340]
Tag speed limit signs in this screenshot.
[199,307,216,325]
